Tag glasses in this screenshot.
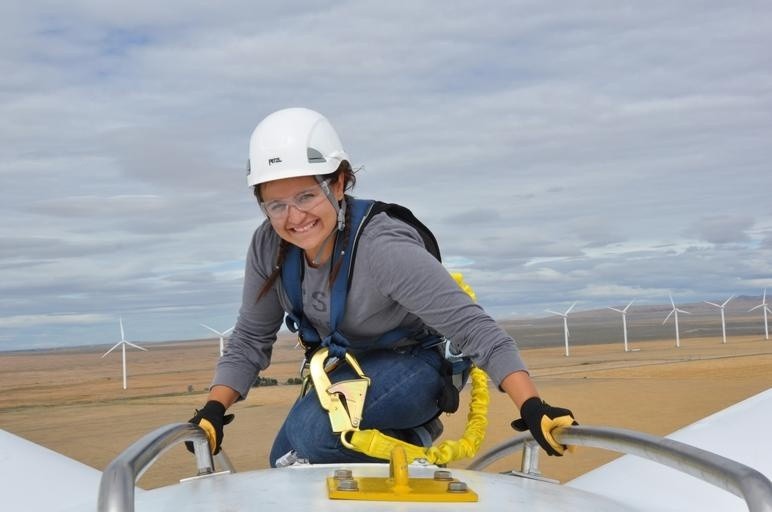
[261,180,330,216]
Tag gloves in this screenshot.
[511,397,579,456]
[184,399,234,454]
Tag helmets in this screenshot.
[246,107,348,186]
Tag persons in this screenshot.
[184,107,580,471]
